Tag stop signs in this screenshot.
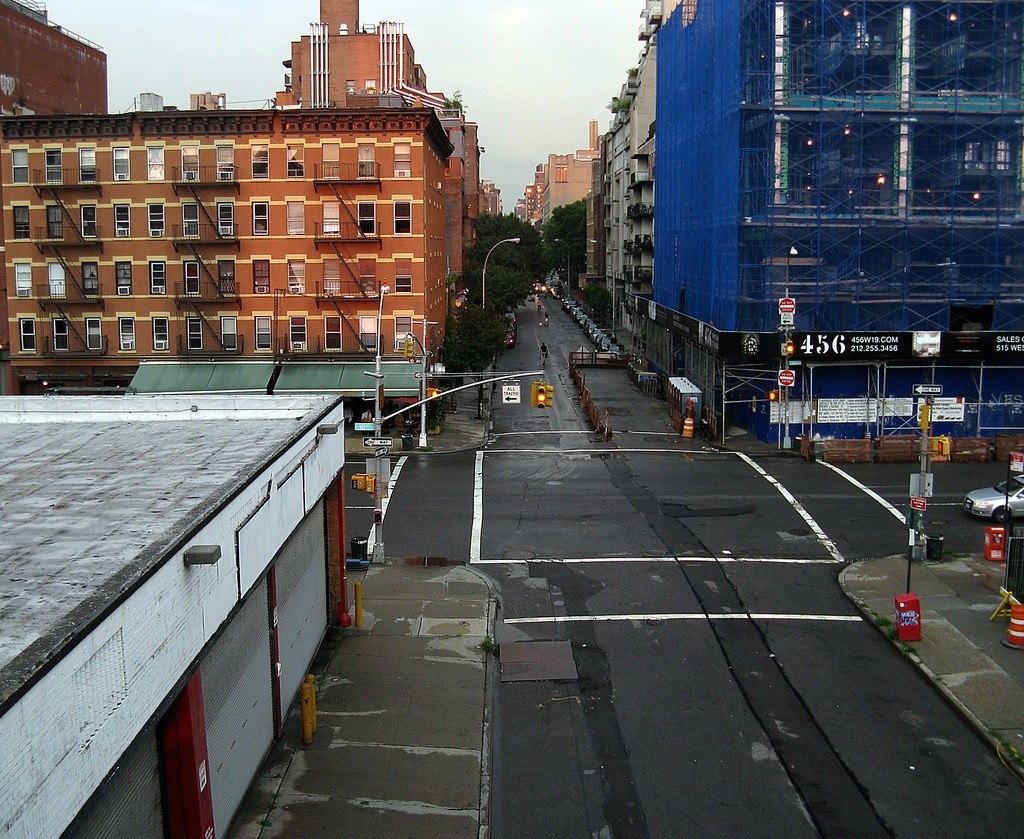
[778,370,796,386]
[778,298,795,314]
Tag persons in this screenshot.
[361,408,372,422]
[537,298,542,311]
[539,342,548,366]
[544,310,549,325]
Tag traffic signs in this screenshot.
[913,384,943,396]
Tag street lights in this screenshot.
[374,284,391,437]
[482,237,521,308]
[555,238,570,297]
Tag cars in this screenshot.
[533,275,630,362]
[962,474,1024,522]
[503,312,518,348]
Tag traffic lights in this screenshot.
[927,437,950,456]
[787,342,794,359]
[426,387,442,400]
[768,391,786,401]
[404,340,414,358]
[537,385,546,409]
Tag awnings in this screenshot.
[273,365,423,397]
[125,363,277,395]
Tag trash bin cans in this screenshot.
[351,537,368,561]
[401,434,413,450]
[925,533,944,559]
